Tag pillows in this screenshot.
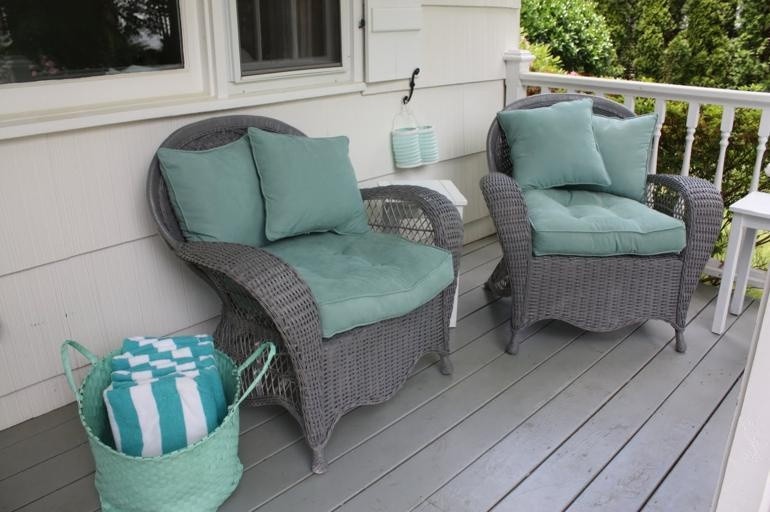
[158,133,272,249]
[246,127,371,239]
[585,112,658,204]
[496,100,612,188]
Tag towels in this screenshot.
[105,335,227,460]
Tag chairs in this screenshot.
[147,93,466,474]
[479,91,727,354]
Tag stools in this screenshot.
[709,189,770,335]
[373,180,468,329]
